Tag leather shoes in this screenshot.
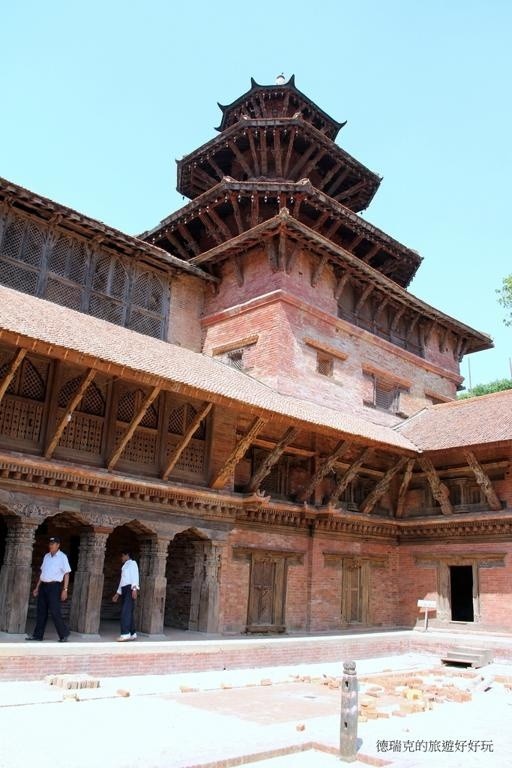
[58,633,70,642]
[25,636,43,640]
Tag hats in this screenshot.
[48,536,60,543]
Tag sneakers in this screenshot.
[116,637,135,641]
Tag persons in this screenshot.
[112,550,140,641]
[25,536,72,642]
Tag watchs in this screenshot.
[63,588,68,592]
[131,589,138,591]
